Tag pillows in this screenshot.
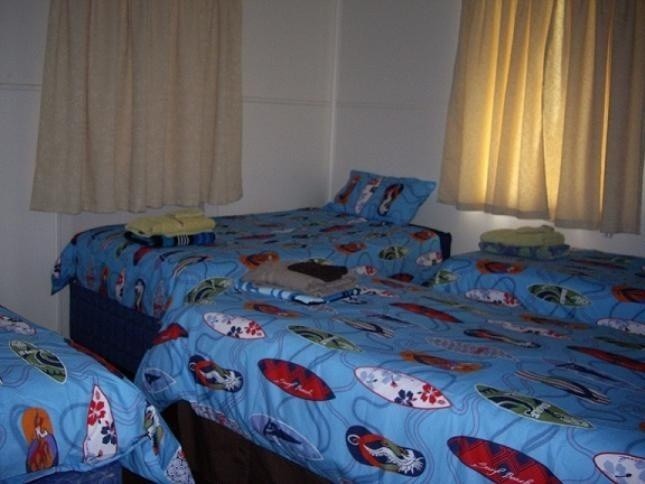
[324,170,437,226]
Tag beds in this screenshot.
[411,247,645,336]
[51,207,452,373]
[0,303,195,484]
[133,270,644,483]
